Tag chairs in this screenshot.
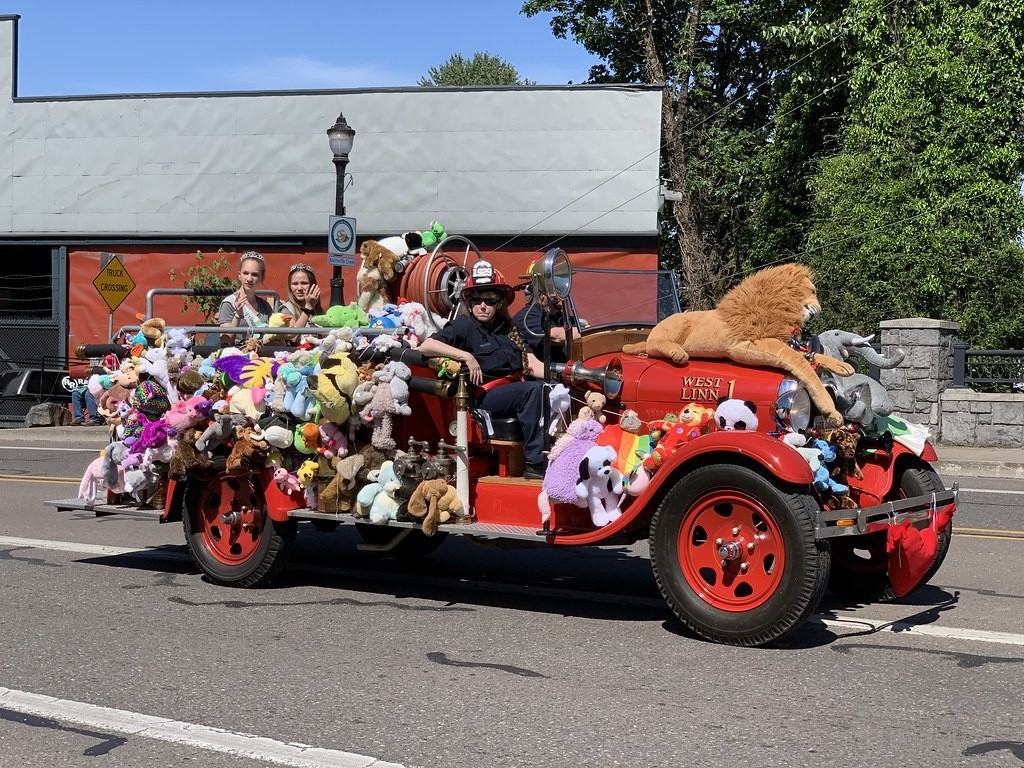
[446,399,524,477]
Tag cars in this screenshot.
[0,367,72,421]
[41,257,960,650]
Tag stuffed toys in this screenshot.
[783,428,864,509]
[314,221,448,352]
[537,384,758,526]
[621,260,855,428]
[817,329,905,422]
[78,318,464,537]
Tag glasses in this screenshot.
[470,296,498,306]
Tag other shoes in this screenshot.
[523,464,544,479]
[87,420,100,426]
[72,418,85,425]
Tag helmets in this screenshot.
[512,253,545,291]
[459,258,515,307]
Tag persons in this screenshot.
[511,284,587,363]
[219,251,273,345]
[278,263,325,347]
[419,259,558,479]
[69,355,107,425]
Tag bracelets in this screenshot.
[300,307,311,313]
[235,314,241,319]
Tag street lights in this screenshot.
[326,111,357,306]
